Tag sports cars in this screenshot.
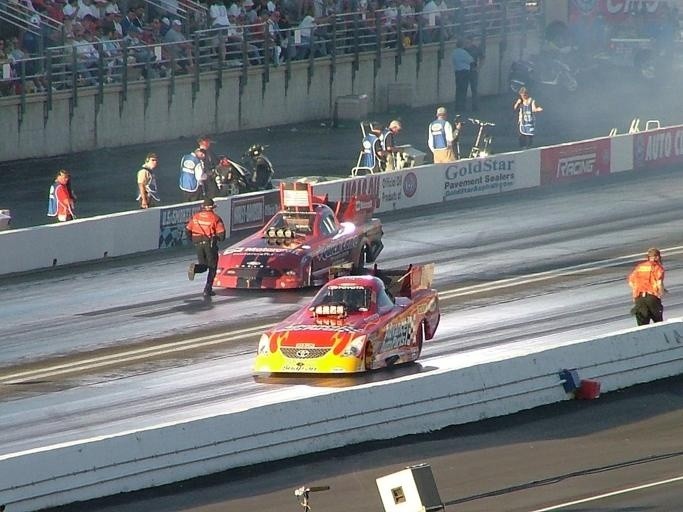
[254,263,448,375]
[206,180,387,290]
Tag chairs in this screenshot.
[0,0,526,95]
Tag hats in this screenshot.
[437,107,447,114]
[389,120,401,129]
[146,152,160,158]
[203,199,214,207]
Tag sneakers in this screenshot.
[188,263,195,280]
[204,292,216,296]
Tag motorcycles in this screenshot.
[210,142,276,195]
[459,115,500,161]
[504,10,682,96]
[290,462,442,512]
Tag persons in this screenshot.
[187,195,226,297]
[46,170,77,222]
[627,247,665,326]
[514,87,543,148]
[361,120,385,174]
[136,152,161,209]
[379,120,405,171]
[427,107,462,164]
[178,149,209,202]
[197,132,222,198]
